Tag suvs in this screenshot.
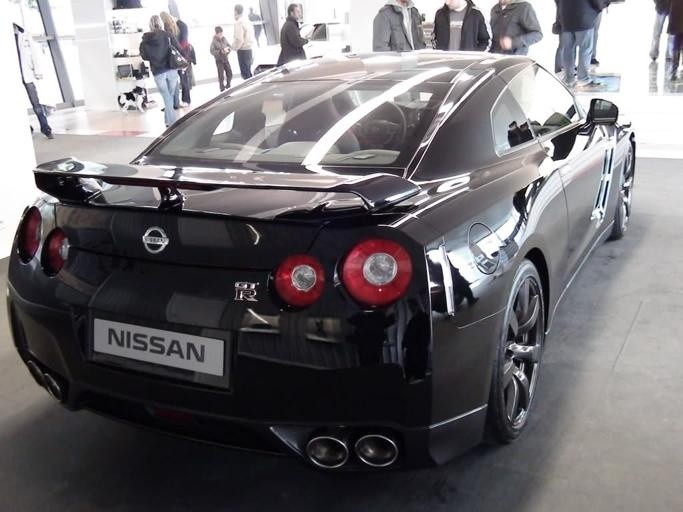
[249,18,351,76]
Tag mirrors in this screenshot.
[529,0,683,93]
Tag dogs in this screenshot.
[117,86,155,114]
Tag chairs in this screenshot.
[280,87,359,154]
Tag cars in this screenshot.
[6,50,638,480]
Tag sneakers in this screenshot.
[580,82,601,89]
[44,130,55,139]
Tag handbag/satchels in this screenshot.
[552,21,561,34]
[168,45,189,69]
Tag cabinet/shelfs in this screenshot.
[113,6,149,81]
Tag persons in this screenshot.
[12,24,53,140]
[554,0,682,90]
[141,11,196,126]
[210,26,232,92]
[430,0,494,51]
[277,4,308,67]
[248,9,261,46]
[372,0,427,51]
[232,5,254,80]
[488,0,542,57]
